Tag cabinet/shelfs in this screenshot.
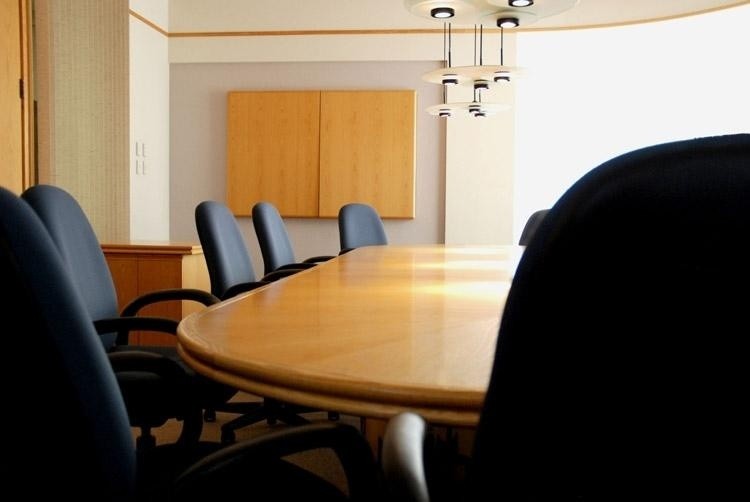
[227,88,417,220]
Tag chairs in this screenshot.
[2,133,750,500]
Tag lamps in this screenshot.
[405,0,578,120]
